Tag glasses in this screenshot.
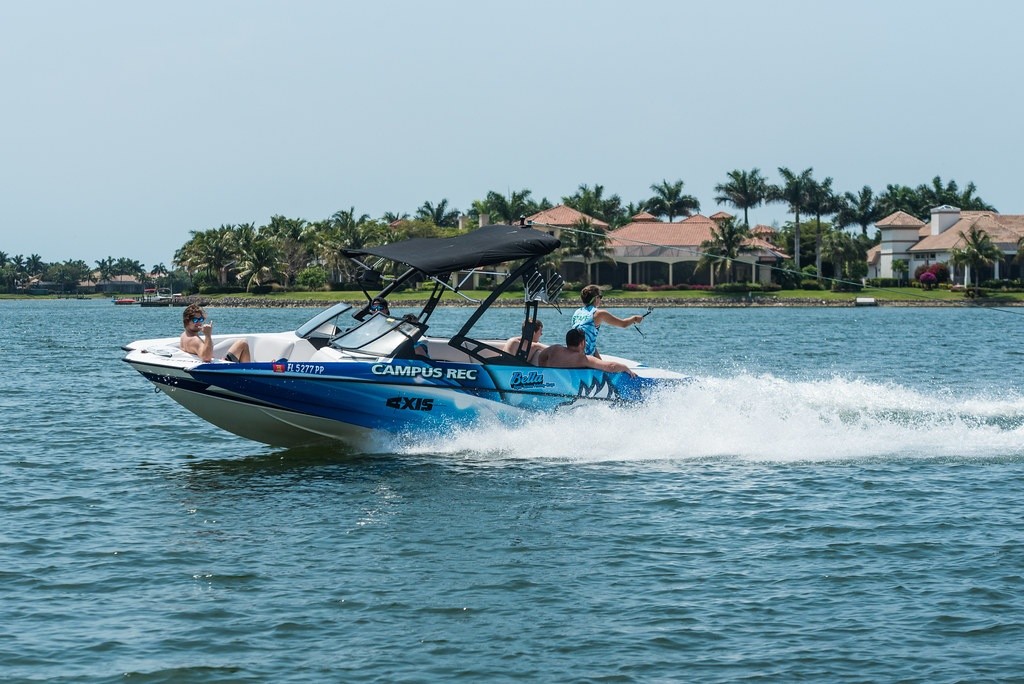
[372,305,384,311]
[590,293,603,303]
[190,316,204,323]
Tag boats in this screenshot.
[117,222,693,461]
[114,298,135,304]
[139,287,172,303]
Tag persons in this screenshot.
[502,318,549,362]
[538,329,638,377]
[372,297,389,316]
[181,305,250,362]
[403,314,429,358]
[574,285,644,360]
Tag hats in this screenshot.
[372,297,390,315]
